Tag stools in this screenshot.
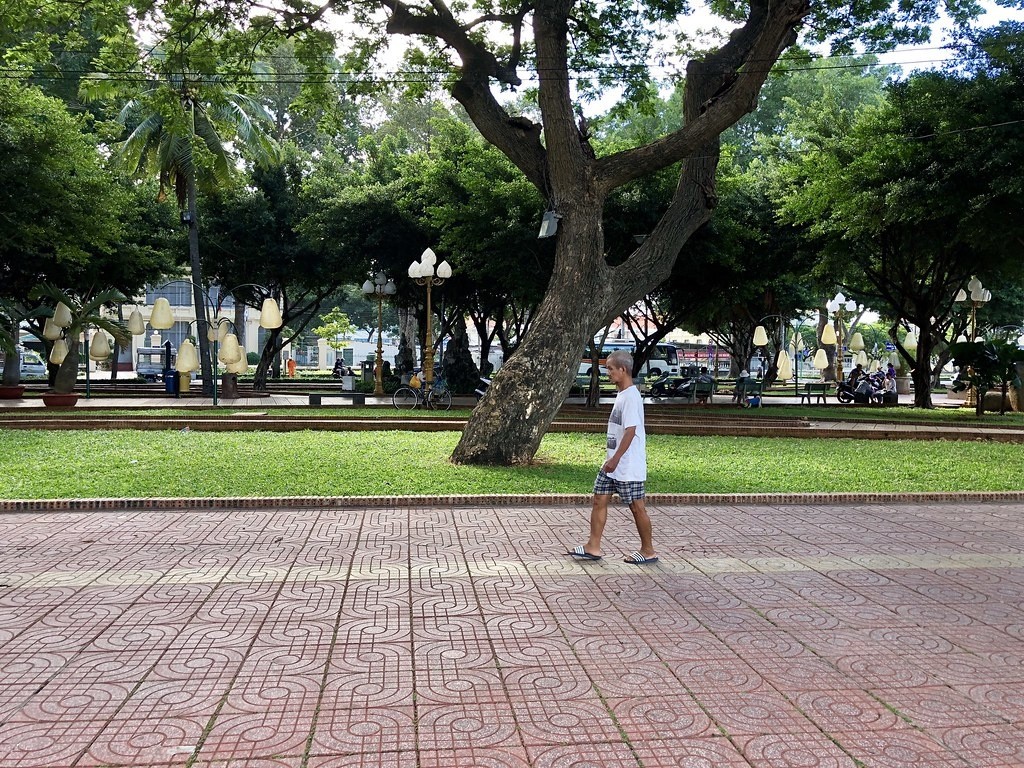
[854,393,869,403]
[883,392,900,404]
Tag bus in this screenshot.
[576,341,685,376]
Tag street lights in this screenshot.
[952,275,992,407]
[362,271,397,397]
[408,247,452,396]
[827,291,857,386]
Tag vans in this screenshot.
[135,347,197,384]
[0,352,47,382]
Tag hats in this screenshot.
[740,370,749,377]
[337,357,340,361]
[341,358,345,361]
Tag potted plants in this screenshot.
[27,283,133,406]
[891,348,917,395]
[0,318,25,401]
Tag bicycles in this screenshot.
[393,369,452,411]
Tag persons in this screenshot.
[480,357,493,377]
[698,367,718,405]
[757,367,762,380]
[288,357,297,378]
[732,369,755,404]
[567,350,658,564]
[847,363,897,405]
[373,359,448,406]
[791,369,796,382]
[335,358,349,377]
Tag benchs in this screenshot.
[309,392,365,404]
[632,378,646,395]
[737,382,765,402]
[578,382,604,397]
[800,382,831,404]
[684,384,714,403]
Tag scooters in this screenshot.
[332,366,357,379]
[650,370,695,398]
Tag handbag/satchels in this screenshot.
[410,374,421,389]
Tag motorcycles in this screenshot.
[836,372,882,404]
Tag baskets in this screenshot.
[401,375,411,385]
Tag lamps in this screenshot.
[537,208,564,239]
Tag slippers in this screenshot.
[567,546,601,560]
[623,551,658,563]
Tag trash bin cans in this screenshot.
[220,373,241,398]
[360,360,375,381]
[165,371,191,392]
[680,366,701,377]
